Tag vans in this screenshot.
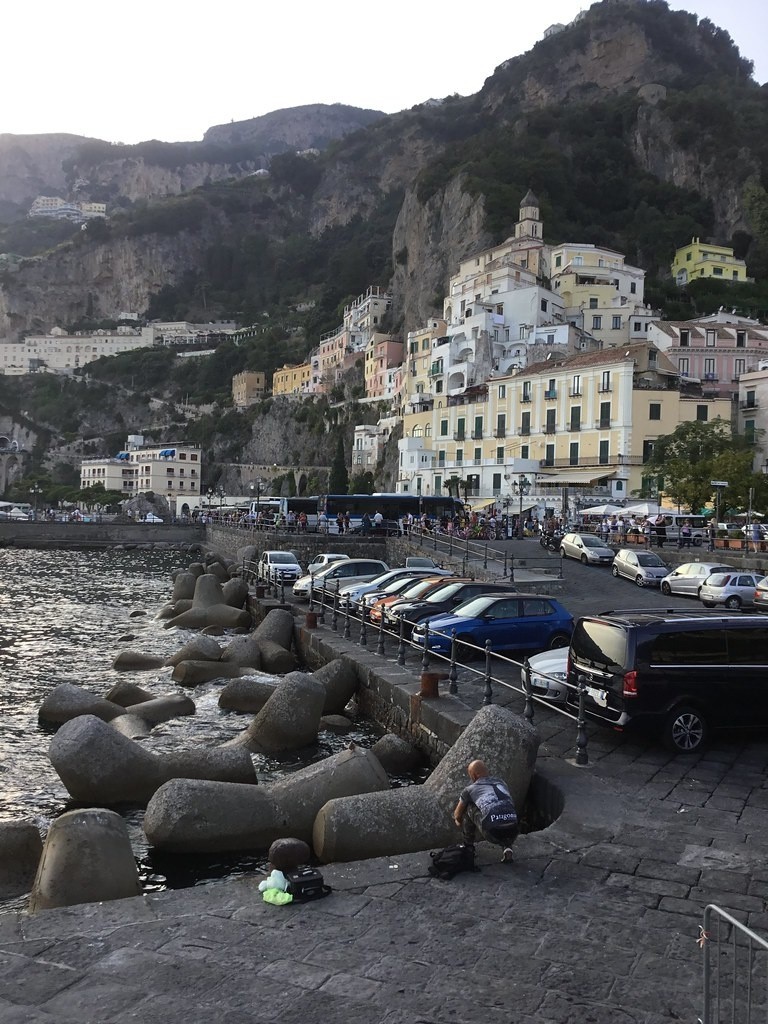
[637,515,708,546]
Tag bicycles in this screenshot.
[456,522,564,553]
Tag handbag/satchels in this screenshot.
[288,868,332,903]
[759,533,765,541]
[428,843,482,879]
[336,518,341,524]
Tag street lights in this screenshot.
[206,487,215,518]
[512,472,531,539]
[213,485,227,524]
[250,475,266,523]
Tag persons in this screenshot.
[171,508,384,537]
[127,507,139,521]
[452,760,519,863]
[398,510,764,554]
[585,281,615,285]
[542,321,552,327]
[0,507,84,522]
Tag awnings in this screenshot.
[470,470,616,515]
[159,449,175,456]
[116,452,129,460]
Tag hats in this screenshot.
[289,511,292,512]
[632,514,636,518]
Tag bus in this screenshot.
[317,493,456,535]
[276,496,322,530]
[251,496,285,525]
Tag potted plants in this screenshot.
[714,529,766,551]
[611,534,646,544]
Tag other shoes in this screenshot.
[501,845,514,863]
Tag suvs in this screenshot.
[382,580,526,641]
[564,606,767,752]
[369,572,481,627]
[356,571,443,621]
[611,549,675,588]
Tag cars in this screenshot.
[706,522,742,538]
[520,645,571,703]
[699,572,765,611]
[559,532,616,565]
[290,559,391,603]
[257,551,303,584]
[660,561,737,597]
[337,563,456,610]
[739,524,768,540]
[409,593,574,664]
[395,556,438,571]
[752,575,768,612]
[306,553,351,576]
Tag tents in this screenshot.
[577,503,682,517]
